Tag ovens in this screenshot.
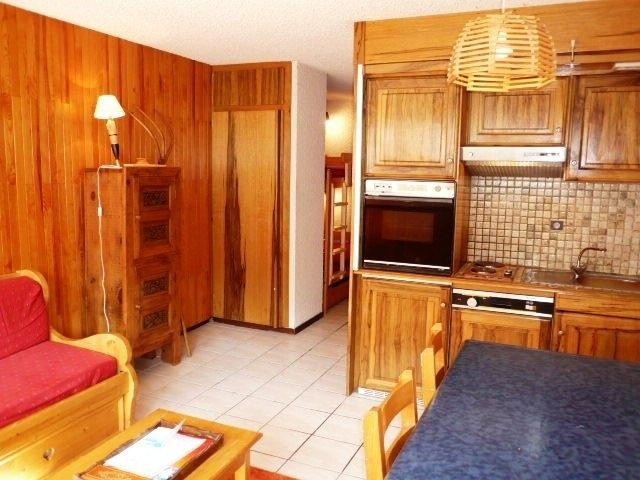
[360,178,456,278]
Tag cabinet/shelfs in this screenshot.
[355,273,640,393]
[363,73,640,183]
[83,166,182,366]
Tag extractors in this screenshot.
[459,145,568,179]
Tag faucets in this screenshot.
[570,246,607,281]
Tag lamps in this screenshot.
[446,1,557,93]
[93,94,126,169]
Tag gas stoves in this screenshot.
[469,260,506,276]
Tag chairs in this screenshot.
[363,322,448,479]
[1,269,140,480]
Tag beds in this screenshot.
[323,152,352,314]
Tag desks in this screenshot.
[382,339,639,480]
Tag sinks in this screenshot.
[533,270,640,294]
